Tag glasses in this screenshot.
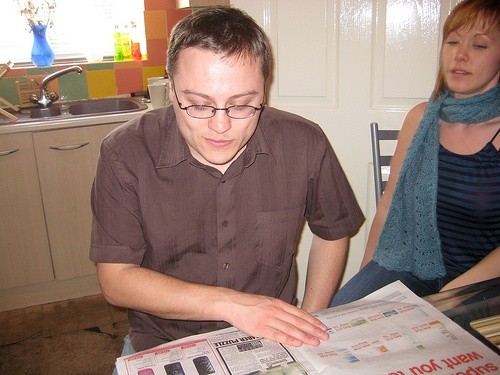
[170,75,266,119]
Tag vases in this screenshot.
[29,24,56,66]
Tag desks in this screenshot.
[115,277,500,375]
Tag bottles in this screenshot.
[122,25,131,60]
[114,25,124,61]
[130,25,141,60]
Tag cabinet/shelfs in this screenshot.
[0,122,129,314]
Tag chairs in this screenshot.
[370,122,400,207]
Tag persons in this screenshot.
[90,5,365,375]
[328,0,500,308]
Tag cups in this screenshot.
[159,79,172,105]
[147,83,166,109]
[147,77,164,84]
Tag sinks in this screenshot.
[0,95,149,129]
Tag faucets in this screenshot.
[29,65,83,107]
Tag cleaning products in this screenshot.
[113,21,143,61]
